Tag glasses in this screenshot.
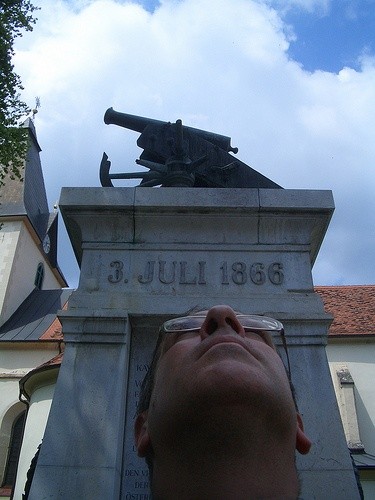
[145,313,301,412]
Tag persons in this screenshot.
[134,305,312,500]
